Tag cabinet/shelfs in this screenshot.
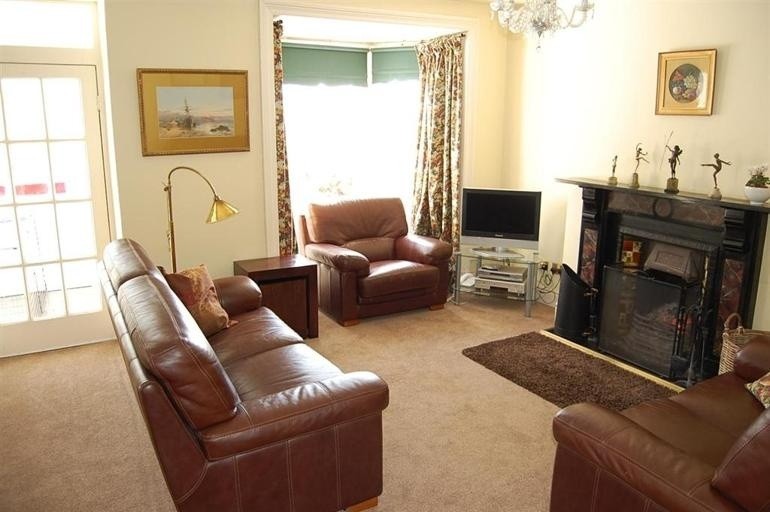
[452,245,544,318]
[233,254,319,339]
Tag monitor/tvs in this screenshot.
[462,188,542,258]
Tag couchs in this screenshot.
[96,237,390,512]
[299,197,453,328]
[549,336,770,512]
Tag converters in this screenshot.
[542,265,547,270]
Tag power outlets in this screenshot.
[552,263,558,273]
[541,261,548,270]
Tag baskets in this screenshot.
[718,313,770,376]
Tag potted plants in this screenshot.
[742,162,770,205]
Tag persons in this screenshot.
[666,145,683,180]
[634,143,650,173]
[701,153,731,189]
[612,155,618,177]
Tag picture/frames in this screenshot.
[655,48,717,116]
[136,67,250,157]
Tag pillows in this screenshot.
[164,264,239,337]
[744,372,769,409]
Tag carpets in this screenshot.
[461,331,677,413]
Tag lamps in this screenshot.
[160,166,239,273]
[490,1,596,54]
